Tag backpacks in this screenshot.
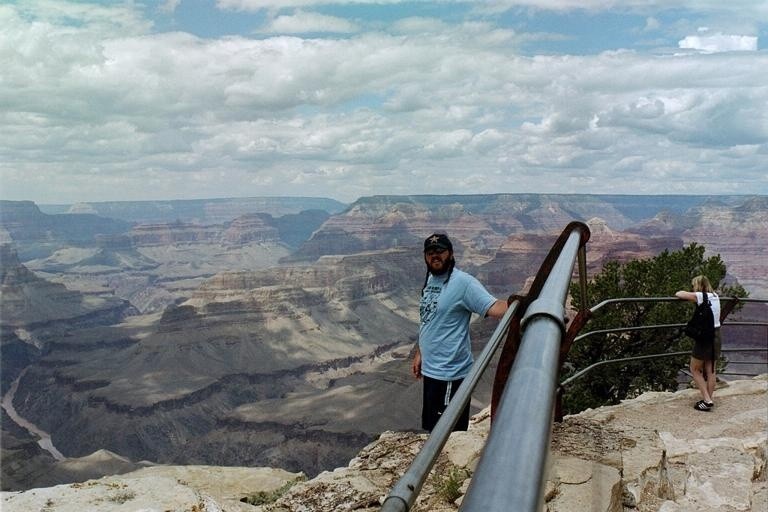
[684,302,713,337]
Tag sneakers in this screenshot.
[694,400,713,410]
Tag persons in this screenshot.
[674,275,722,412]
[409,232,510,435]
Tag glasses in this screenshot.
[427,247,447,255]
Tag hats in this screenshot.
[424,234,452,251]
